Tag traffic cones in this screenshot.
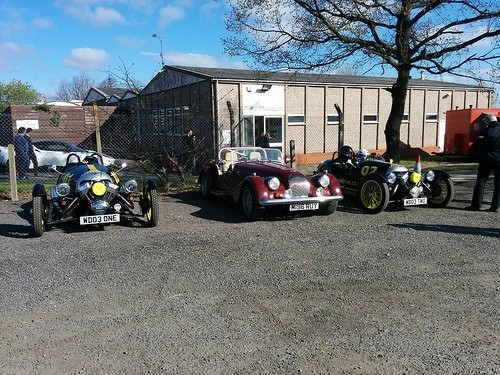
[414,154,421,174]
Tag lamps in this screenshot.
[261,84,273,92]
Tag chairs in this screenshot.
[223,151,239,174]
[247,151,262,160]
[339,145,357,169]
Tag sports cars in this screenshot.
[0,139,114,171]
[194,147,344,221]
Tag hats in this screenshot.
[484,114,497,124]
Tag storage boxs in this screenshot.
[442,107,500,155]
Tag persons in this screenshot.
[14,127,30,181]
[465,113,500,212]
[255,131,270,148]
[23,128,40,177]
[358,149,368,161]
[184,131,196,170]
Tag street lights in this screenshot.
[152,33,163,69]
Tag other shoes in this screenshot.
[465,206,480,211]
[485,206,499,212]
